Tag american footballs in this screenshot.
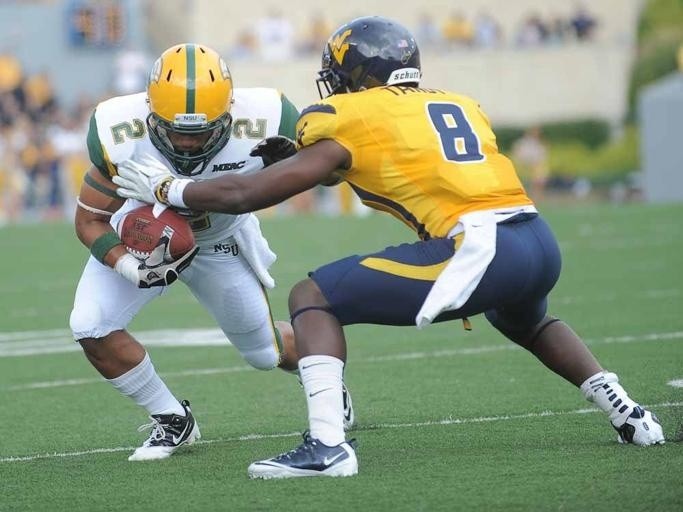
[118,205,195,264]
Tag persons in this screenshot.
[411,5,594,48]
[510,123,547,200]
[67,39,362,462]
[0,55,92,213]
[106,18,672,485]
[245,5,335,62]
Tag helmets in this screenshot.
[316,14,423,100]
[145,41,234,177]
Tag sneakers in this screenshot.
[127,393,201,465]
[246,430,358,483]
[606,406,666,450]
[293,373,355,433]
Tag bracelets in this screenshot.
[89,229,124,266]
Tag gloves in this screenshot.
[111,153,175,219]
[136,239,202,292]
[250,135,296,169]
